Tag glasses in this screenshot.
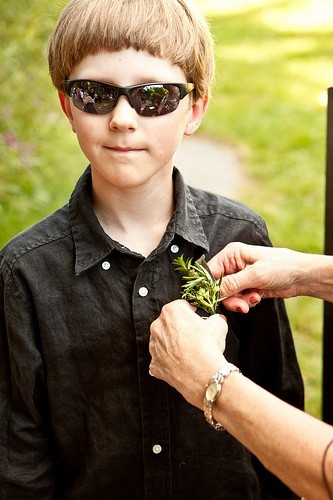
[63,79,195,117]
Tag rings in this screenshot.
[148,363,156,377]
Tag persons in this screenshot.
[0,0,307,500]
[131,86,167,116]
[70,82,114,114]
[147,242,333,500]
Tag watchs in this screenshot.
[202,362,242,431]
